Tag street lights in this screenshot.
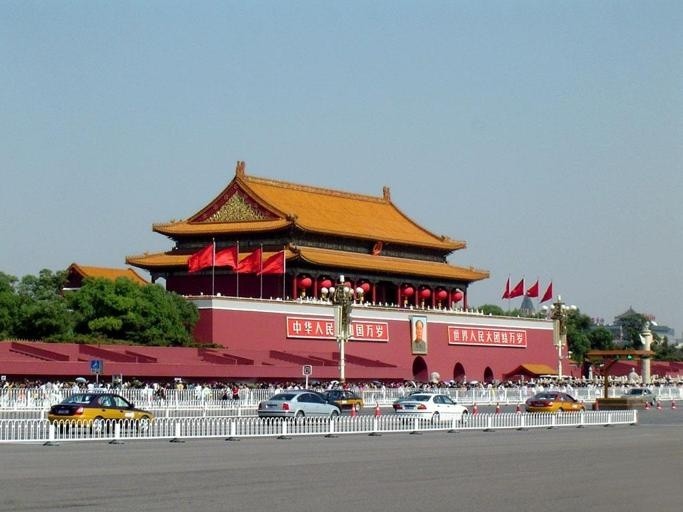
[542,295,576,379]
[321,275,364,382]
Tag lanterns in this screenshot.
[297,275,462,307]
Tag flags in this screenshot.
[187,241,213,272]
[256,252,284,276]
[540,283,552,304]
[510,279,523,298]
[502,279,509,300]
[527,282,538,297]
[232,249,261,274]
[215,245,237,268]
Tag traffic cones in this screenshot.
[657,400,661,410]
[595,401,599,411]
[515,404,521,415]
[473,405,478,415]
[671,400,675,409]
[644,401,649,409]
[350,402,356,416]
[495,404,500,414]
[375,404,381,416]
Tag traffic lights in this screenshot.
[627,355,632,360]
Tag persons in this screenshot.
[412,320,426,352]
[0,377,683,400]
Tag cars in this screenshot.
[322,390,363,412]
[393,390,469,424]
[621,388,657,407]
[526,391,585,417]
[258,389,341,426]
[49,393,153,435]
